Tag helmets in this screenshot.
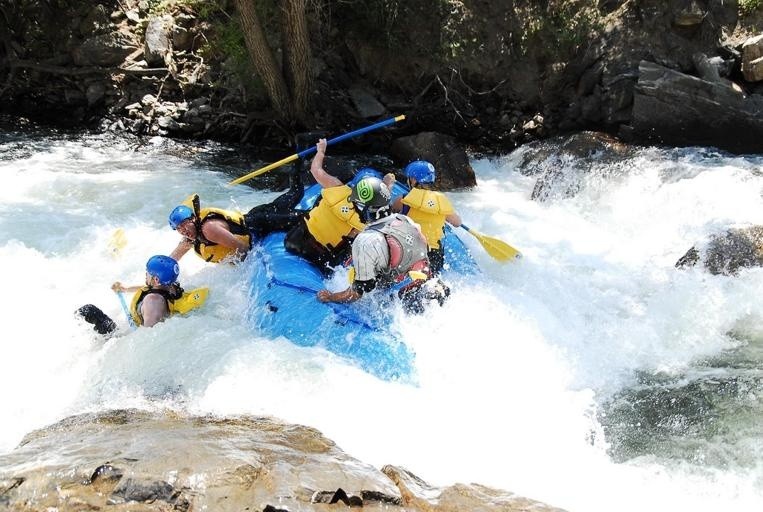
[347,168,382,189]
[347,176,392,207]
[146,255,180,285]
[403,160,436,186]
[168,205,195,230]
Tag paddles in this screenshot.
[392,180,522,265]
[228,114,406,184]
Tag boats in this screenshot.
[238,175,478,383]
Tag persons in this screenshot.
[75,255,183,339]
[381,157,464,277]
[314,177,449,317]
[168,161,314,264]
[283,137,391,280]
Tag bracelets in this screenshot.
[318,150,324,154]
